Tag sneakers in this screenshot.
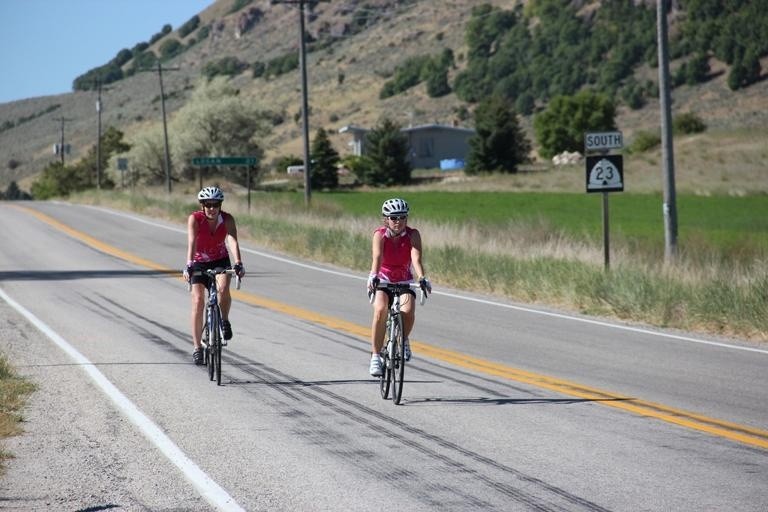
[191,348,203,364]
[399,339,410,361]
[369,357,383,376]
[221,320,232,340]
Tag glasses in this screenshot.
[203,202,220,208]
[387,216,406,220]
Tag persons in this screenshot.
[366,197,432,377]
[182,186,246,367]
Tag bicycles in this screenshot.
[370,277,427,405]
[188,266,242,386]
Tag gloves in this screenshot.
[419,276,431,293]
[182,264,193,279]
[235,262,245,278]
[366,274,377,298]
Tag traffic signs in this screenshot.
[192,156,257,166]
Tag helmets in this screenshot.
[381,198,409,217]
[197,186,223,201]
